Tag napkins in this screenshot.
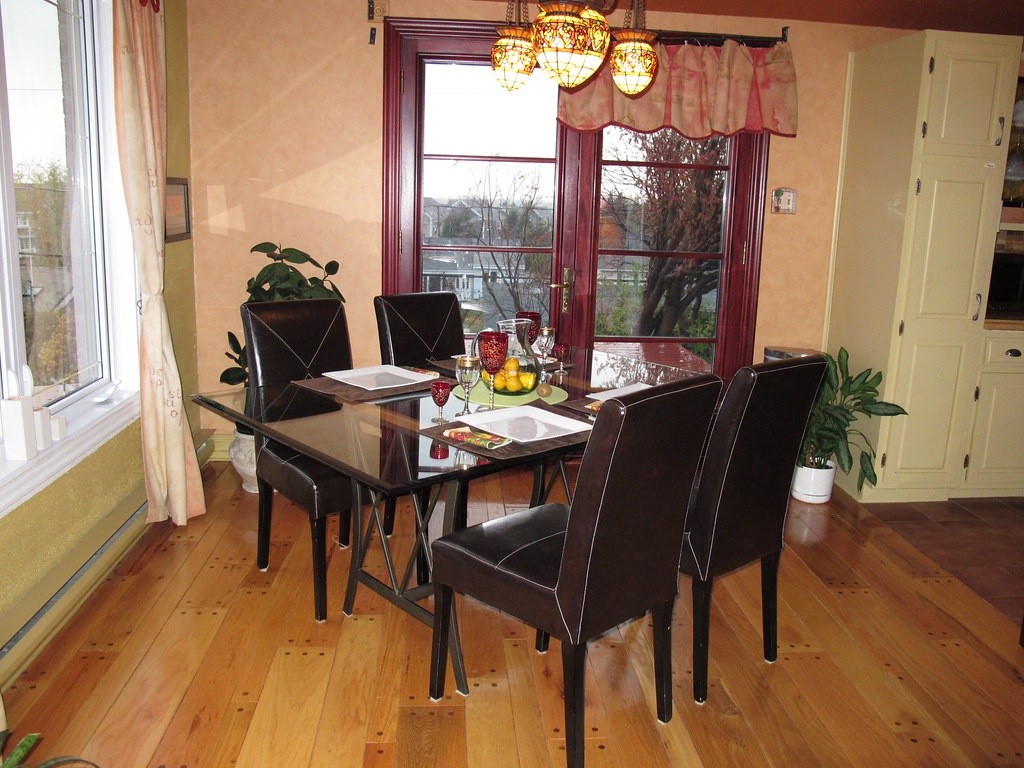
[583,400,603,413]
[442,426,513,450]
[399,366,440,379]
[534,353,558,362]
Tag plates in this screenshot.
[451,354,558,366]
[321,364,437,391]
[452,384,569,409]
[455,402,595,444]
[585,382,654,402]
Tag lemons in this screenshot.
[482,358,536,392]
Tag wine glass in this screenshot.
[476,331,509,412]
[430,423,450,459]
[515,310,541,367]
[552,342,570,375]
[535,325,556,379]
[430,381,451,423]
[454,356,480,417]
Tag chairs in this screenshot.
[374,291,467,534]
[428,374,723,768]
[636,355,829,705]
[241,298,428,624]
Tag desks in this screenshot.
[187,342,729,697]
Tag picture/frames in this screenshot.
[165,176,192,243]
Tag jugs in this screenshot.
[471,318,541,395]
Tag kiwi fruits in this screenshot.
[536,384,552,397]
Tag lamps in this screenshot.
[491,0,660,97]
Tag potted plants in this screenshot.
[790,347,909,504]
[226,242,347,493]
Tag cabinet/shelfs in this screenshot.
[822,28,1024,504]
